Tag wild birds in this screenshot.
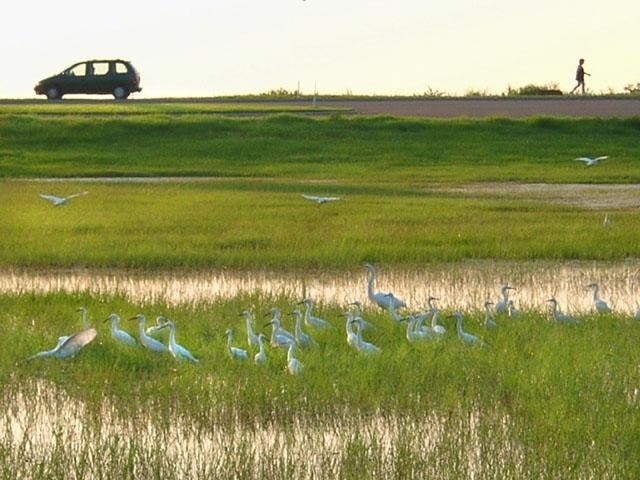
[574,155,609,166]
[337,299,382,358]
[585,283,612,318]
[223,296,328,374]
[483,285,520,329]
[360,262,406,323]
[543,297,578,322]
[445,312,483,347]
[399,296,447,344]
[21,305,200,368]
[38,190,90,206]
[300,192,341,203]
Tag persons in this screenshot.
[571,58,591,93]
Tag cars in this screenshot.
[34,60,142,99]
